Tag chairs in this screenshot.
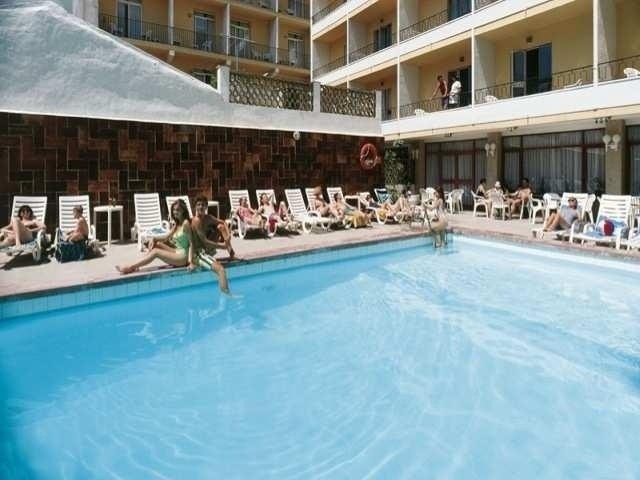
[565,77,582,89]
[412,105,427,117]
[623,65,640,79]
[223,183,433,240]
[441,184,640,260]
[0,188,199,268]
[485,91,497,104]
[103,17,301,67]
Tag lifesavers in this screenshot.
[360,144,378,170]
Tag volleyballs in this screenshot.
[598,220,614,236]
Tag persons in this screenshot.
[448,75,462,110]
[234,196,266,229]
[64,205,88,243]
[475,178,532,220]
[429,75,449,110]
[257,193,295,232]
[544,197,581,231]
[0,205,47,250]
[311,179,448,248]
[191,195,242,294]
[115,199,194,275]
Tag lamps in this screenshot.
[600,131,623,157]
[483,140,499,159]
